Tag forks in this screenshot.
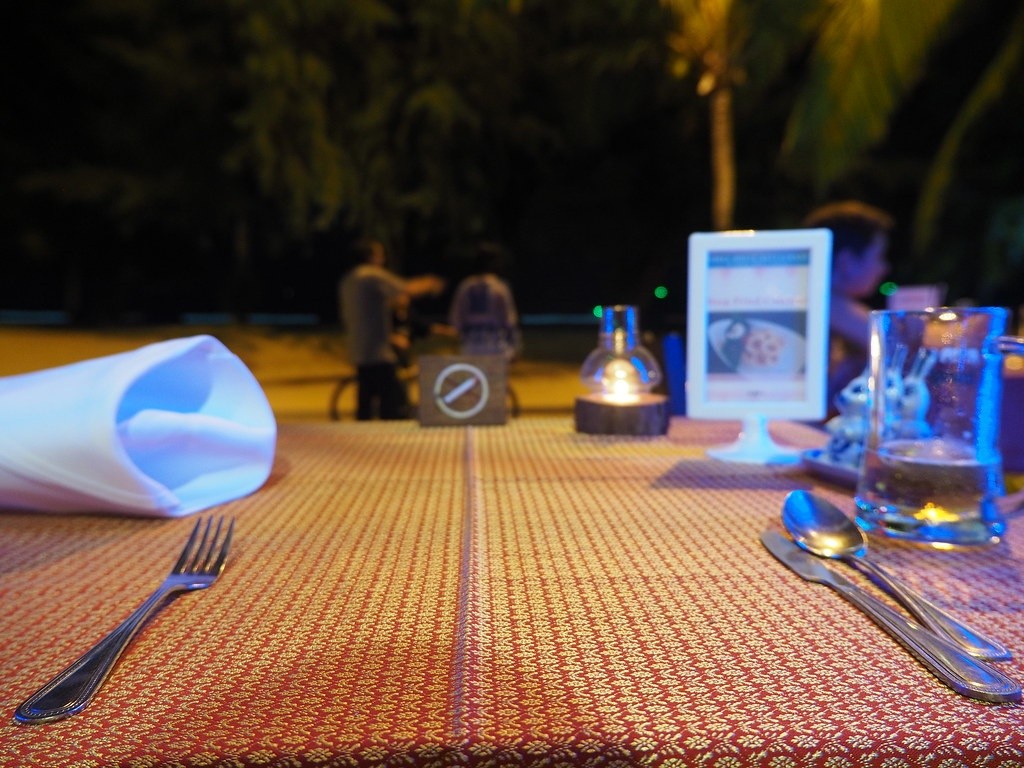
[10,514,236,723]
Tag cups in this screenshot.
[851,305,1024,544]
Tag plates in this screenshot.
[708,318,805,375]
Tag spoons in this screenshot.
[780,489,1013,661]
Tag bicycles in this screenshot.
[328,325,520,425]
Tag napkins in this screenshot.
[0,336,285,517]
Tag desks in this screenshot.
[0,337,1024,768]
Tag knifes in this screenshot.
[759,530,1021,702]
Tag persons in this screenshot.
[335,239,444,421]
[797,201,993,428]
[446,244,520,371]
